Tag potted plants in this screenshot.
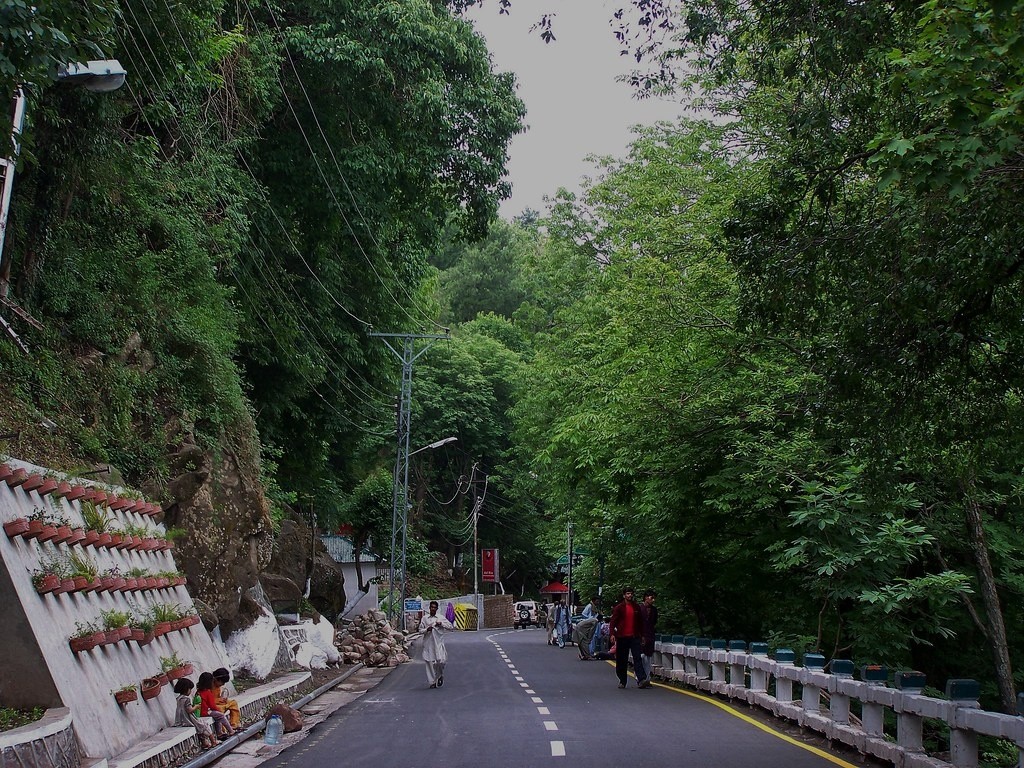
[24,548,71,597]
[141,677,161,700]
[141,570,157,594]
[0,454,187,553]
[153,668,170,687]
[176,572,189,585]
[120,571,137,594]
[46,548,76,596]
[153,569,166,591]
[179,658,193,677]
[66,545,102,596]
[129,567,150,593]
[160,649,186,682]
[68,569,94,597]
[107,560,126,594]
[67,597,201,653]
[169,573,180,588]
[91,554,115,594]
[163,571,175,589]
[109,683,139,705]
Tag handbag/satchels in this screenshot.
[265,704,303,734]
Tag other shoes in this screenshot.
[548,642,552,645]
[203,743,212,750]
[233,728,241,733]
[217,733,228,740]
[437,676,443,686]
[560,647,564,649]
[617,681,626,688]
[638,680,653,689]
[230,730,238,735]
[430,683,436,689]
[212,740,223,747]
[237,727,248,732]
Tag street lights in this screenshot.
[0,56,129,276]
[387,436,458,622]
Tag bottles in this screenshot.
[264,715,283,745]
[193,693,202,717]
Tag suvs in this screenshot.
[513,601,548,630]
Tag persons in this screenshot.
[194,672,243,740]
[209,668,247,735]
[419,601,453,690]
[541,587,658,688]
[174,678,223,750]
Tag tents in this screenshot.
[540,581,573,606]
